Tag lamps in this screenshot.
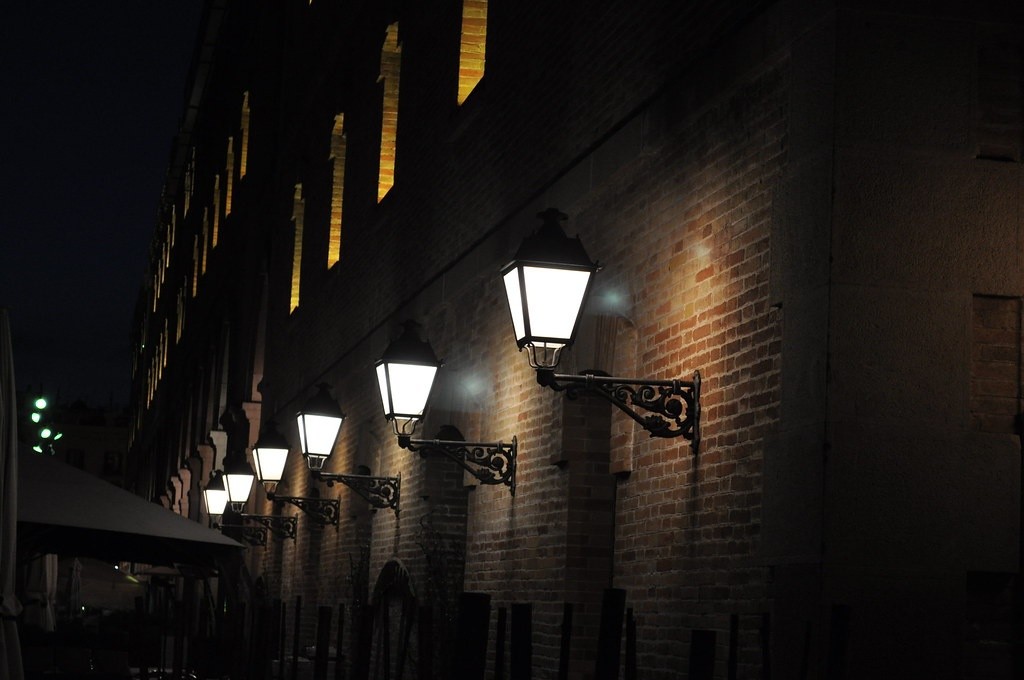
[500,206,701,459]
[127,572,140,583]
[369,316,517,500]
[295,379,400,518]
[221,450,299,546]
[249,416,340,535]
[203,468,267,552]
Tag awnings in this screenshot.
[15,445,245,568]
